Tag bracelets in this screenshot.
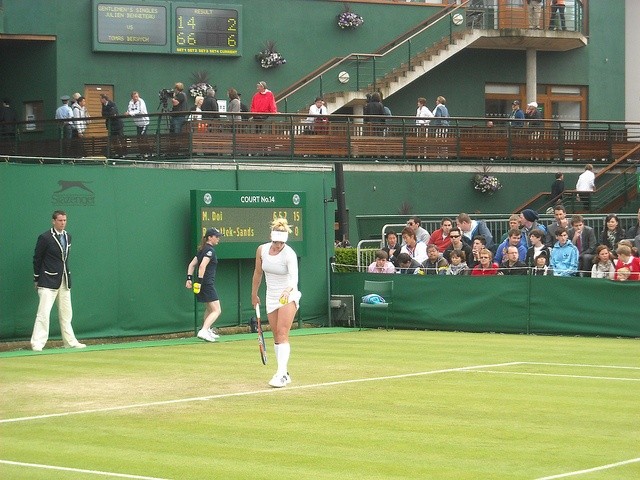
[196,278,203,283]
[186,275,193,280]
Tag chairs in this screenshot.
[359,280,394,331]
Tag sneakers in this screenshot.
[269,373,288,387]
[197,329,215,342]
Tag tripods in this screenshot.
[150,108,170,161]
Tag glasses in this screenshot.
[449,235,460,238]
[480,256,489,259]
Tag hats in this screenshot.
[522,209,539,222]
[271,230,288,242]
[206,228,224,236]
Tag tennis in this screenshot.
[279,297,286,304]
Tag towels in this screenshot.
[361,293,385,304]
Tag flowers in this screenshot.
[187,82,216,100]
[255,50,286,69]
[472,173,502,193]
[337,12,363,30]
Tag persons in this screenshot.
[615,246,640,280]
[399,227,429,265]
[617,239,637,256]
[528,0,543,30]
[575,164,596,209]
[568,215,596,276]
[402,217,431,246]
[432,96,451,159]
[55,95,73,138]
[186,95,204,120]
[422,244,449,275]
[367,250,396,274]
[238,93,248,121]
[530,255,552,276]
[550,227,578,276]
[546,205,572,247]
[70,93,91,125]
[250,81,281,121]
[99,93,124,137]
[496,245,528,275]
[598,213,625,251]
[184,228,223,342]
[30,210,86,351]
[427,218,453,253]
[508,99,525,128]
[446,250,469,275]
[494,229,527,266]
[415,98,433,159]
[470,250,498,276]
[71,97,87,138]
[499,214,521,244]
[306,97,329,134]
[226,89,242,122]
[365,92,386,136]
[169,83,188,135]
[590,244,616,281]
[381,231,402,271]
[548,0,568,31]
[456,213,493,249]
[467,235,486,268]
[626,208,640,238]
[519,209,548,246]
[201,89,220,120]
[396,253,424,275]
[443,227,473,267]
[382,107,392,136]
[525,229,549,275]
[551,172,564,209]
[125,91,151,159]
[250,219,302,389]
[525,101,544,160]
